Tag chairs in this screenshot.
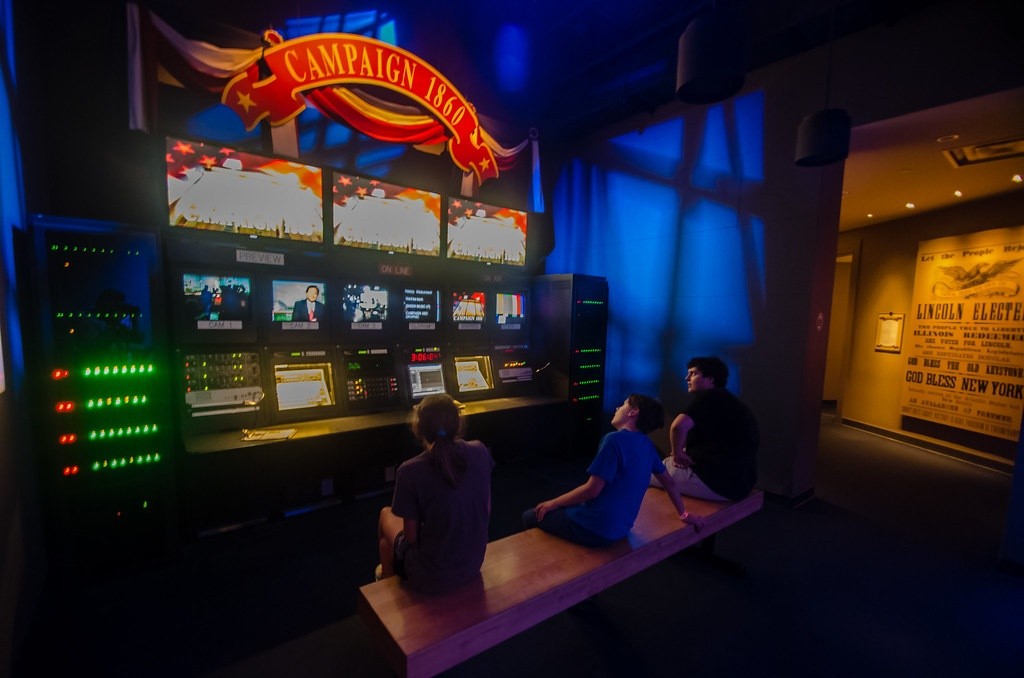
[378,305,385,320]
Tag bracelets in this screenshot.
[679,511,688,520]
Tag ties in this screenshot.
[309,303,314,320]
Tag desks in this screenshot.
[359,303,379,321]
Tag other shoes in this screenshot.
[374,563,383,581]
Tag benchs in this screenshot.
[359,485,764,678]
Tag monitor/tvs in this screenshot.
[157,132,534,421]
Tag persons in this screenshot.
[375,392,496,594]
[523,396,706,548]
[292,286,323,323]
[651,357,758,502]
